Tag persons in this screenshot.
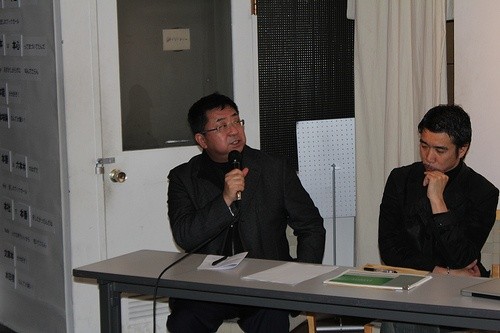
[378,104,499,332]
[166,93,326,333]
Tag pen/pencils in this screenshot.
[212,254,227,265]
[364,267,398,273]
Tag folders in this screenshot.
[461,276,500,299]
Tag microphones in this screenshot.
[228,150,242,200]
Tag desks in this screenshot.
[72,250,500,333]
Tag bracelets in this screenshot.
[448,268,450,274]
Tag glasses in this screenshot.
[200,118,244,134]
